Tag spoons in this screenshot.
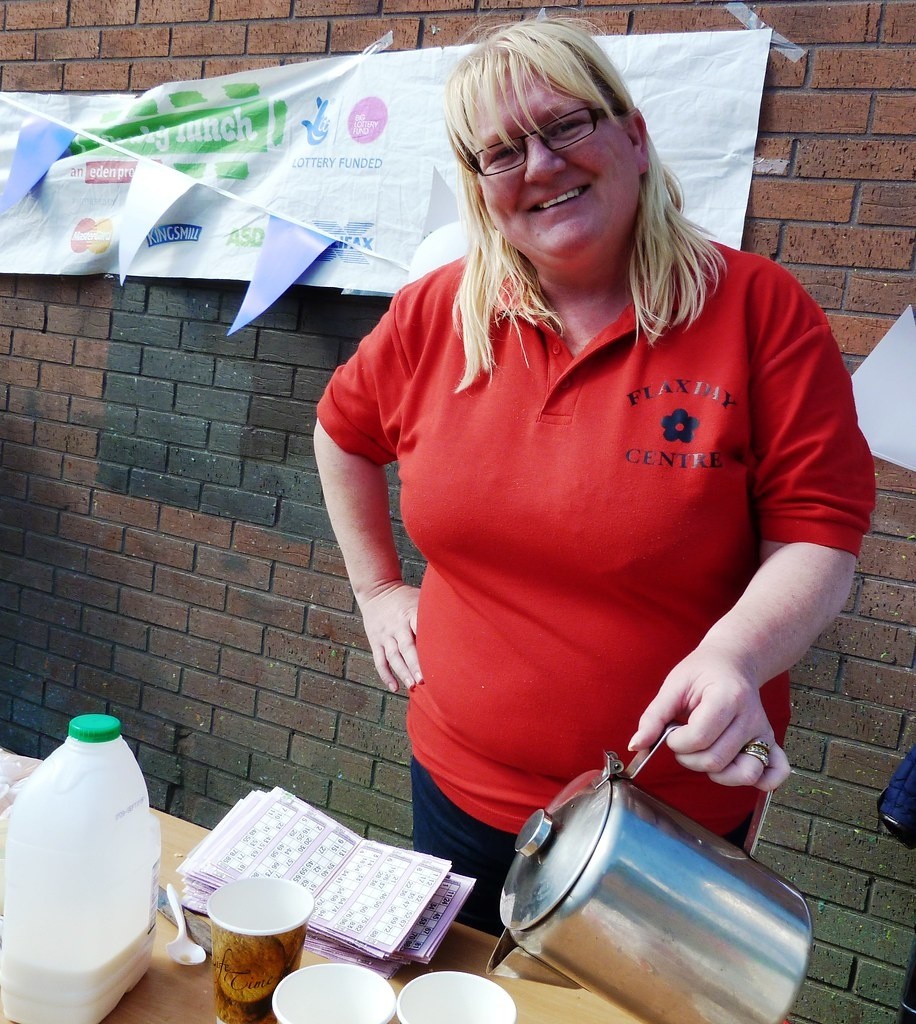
[165,884,206,966]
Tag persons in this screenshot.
[312,18,879,935]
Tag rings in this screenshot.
[744,740,770,768]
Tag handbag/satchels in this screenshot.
[879,744,916,849]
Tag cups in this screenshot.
[206,877,316,1024]
[396,970,518,1024]
[271,963,395,1024]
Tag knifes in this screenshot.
[156,883,212,954]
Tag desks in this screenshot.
[99,801,656,1023]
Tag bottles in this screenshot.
[0,713,161,1024]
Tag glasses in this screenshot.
[469,106,626,177]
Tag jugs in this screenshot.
[485,726,811,1024]
